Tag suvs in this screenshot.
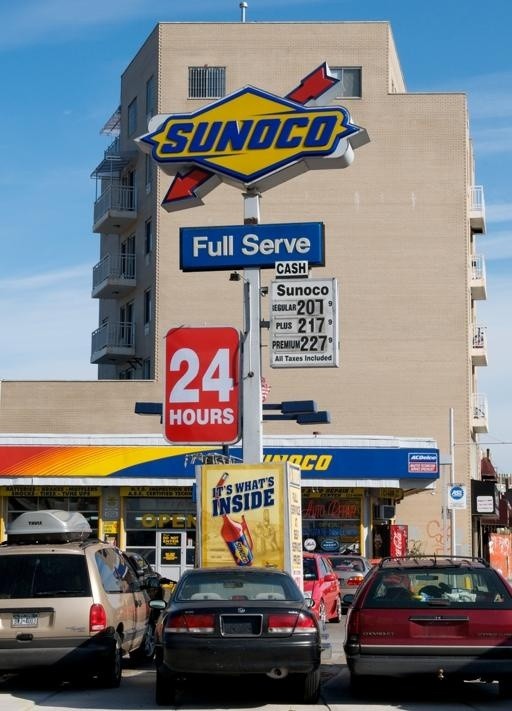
[0,507,164,691]
